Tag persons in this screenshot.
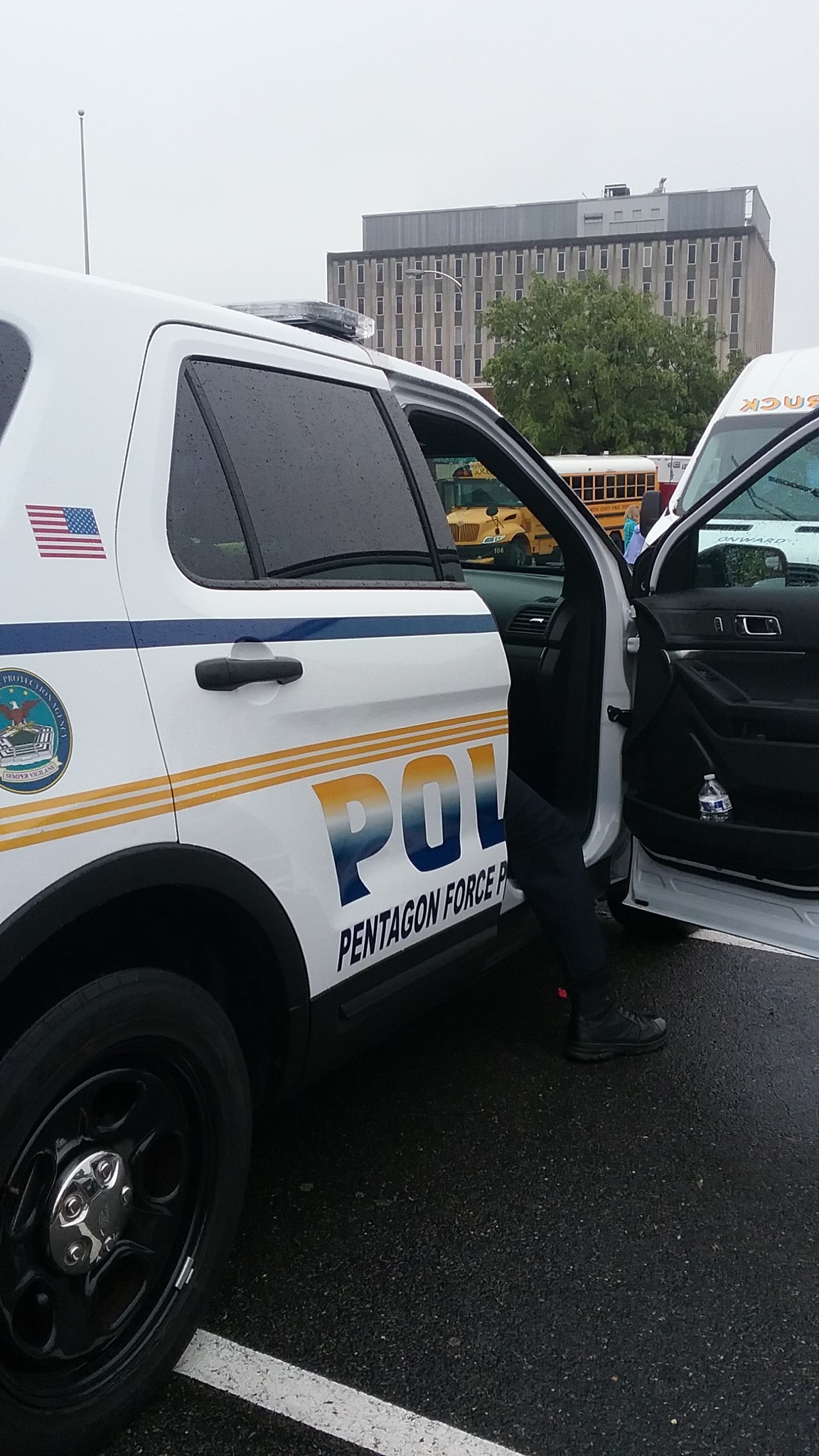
[624,522,646,571]
[501,769,669,1062]
[623,505,641,553]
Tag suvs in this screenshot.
[0,260,819,1456]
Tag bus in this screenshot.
[442,453,662,568]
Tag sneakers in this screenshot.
[563,1001,669,1061]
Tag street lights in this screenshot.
[404,268,470,387]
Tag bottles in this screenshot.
[698,773,733,823]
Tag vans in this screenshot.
[647,351,819,586]
